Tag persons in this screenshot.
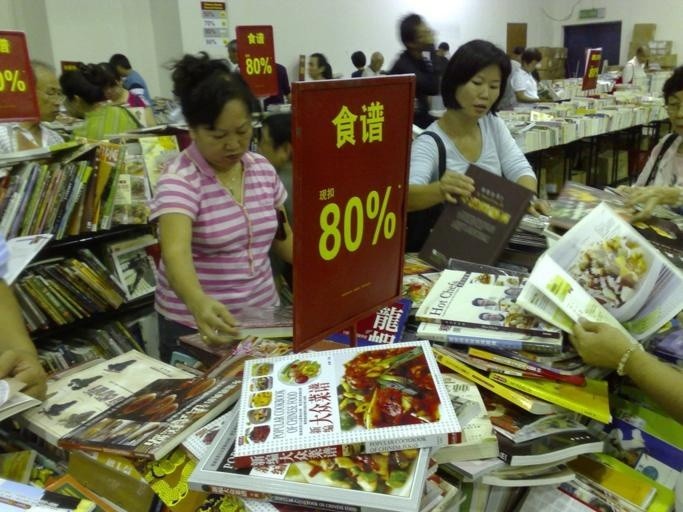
[0,13,547,399]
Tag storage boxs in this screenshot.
[535,134,652,199]
[534,46,568,80]
[628,24,676,72]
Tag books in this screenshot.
[0,62,681,512]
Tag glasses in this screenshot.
[662,104,679,112]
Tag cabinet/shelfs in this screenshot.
[29,225,154,348]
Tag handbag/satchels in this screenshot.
[405,203,444,253]
